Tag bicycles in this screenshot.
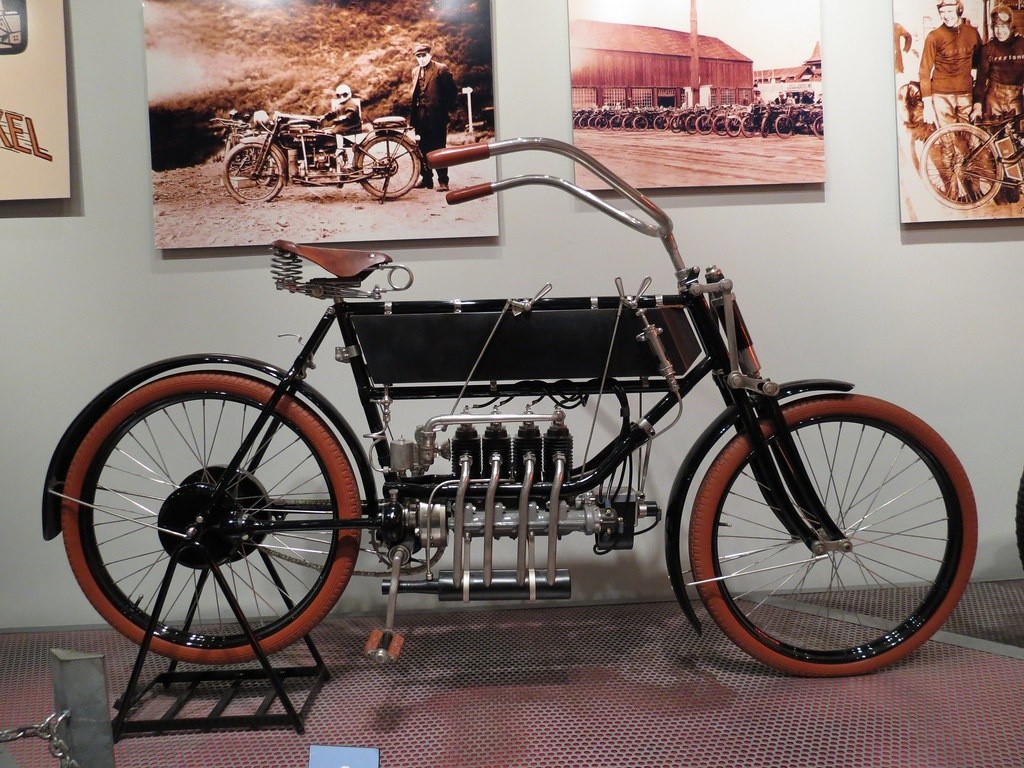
[918,108,1024,211]
[572,104,823,141]
[38,134,980,736]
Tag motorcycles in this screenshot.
[208,110,431,207]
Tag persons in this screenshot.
[969,2,1024,184]
[409,44,458,191]
[893,0,1021,197]
[316,84,362,159]
[742,88,815,106]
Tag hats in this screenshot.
[413,44,431,56]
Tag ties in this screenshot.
[420,67,425,78]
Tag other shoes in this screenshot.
[412,178,433,189]
[964,175,981,200]
[437,182,448,191]
[944,171,959,199]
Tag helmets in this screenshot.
[991,4,1012,27]
[335,84,351,104]
[937,0,963,16]
